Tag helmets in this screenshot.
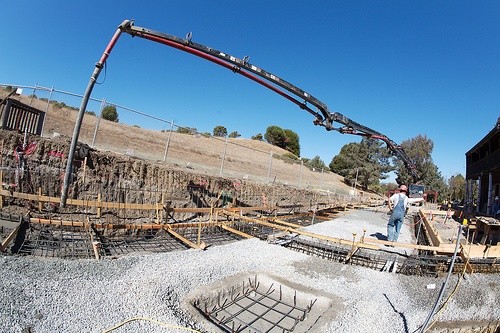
[400,184,407,191]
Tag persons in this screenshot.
[387,184,409,247]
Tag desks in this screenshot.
[475,215,500,246]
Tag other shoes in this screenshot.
[388,244,395,247]
